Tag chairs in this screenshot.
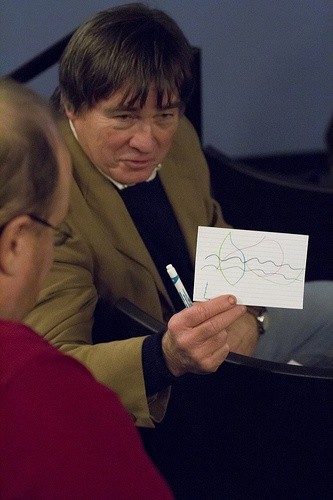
[1,29,333,500]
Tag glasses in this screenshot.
[27,213,74,247]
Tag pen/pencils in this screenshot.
[164,264,192,309]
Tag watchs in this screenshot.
[246,305,270,336]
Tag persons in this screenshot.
[0,78,175,499]
[23,2,331,431]
